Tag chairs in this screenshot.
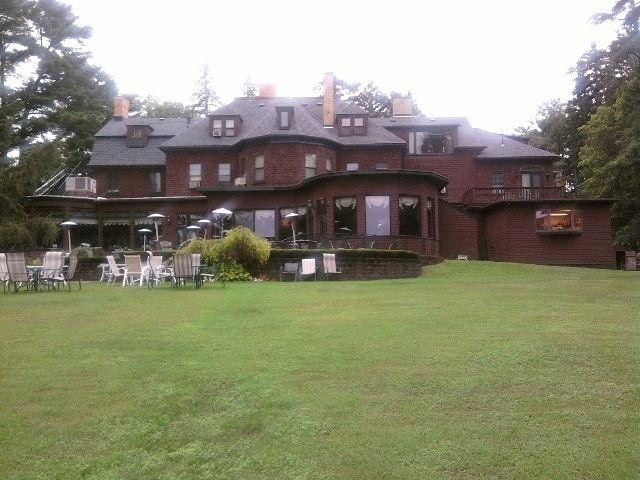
[98,253,227,290]
[279,253,344,282]
[0,251,82,293]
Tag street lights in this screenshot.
[60,221,77,253]
[285,213,299,245]
[137,214,166,251]
[186,208,233,239]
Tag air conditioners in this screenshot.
[234,177,246,186]
[64,176,96,194]
[212,130,221,137]
